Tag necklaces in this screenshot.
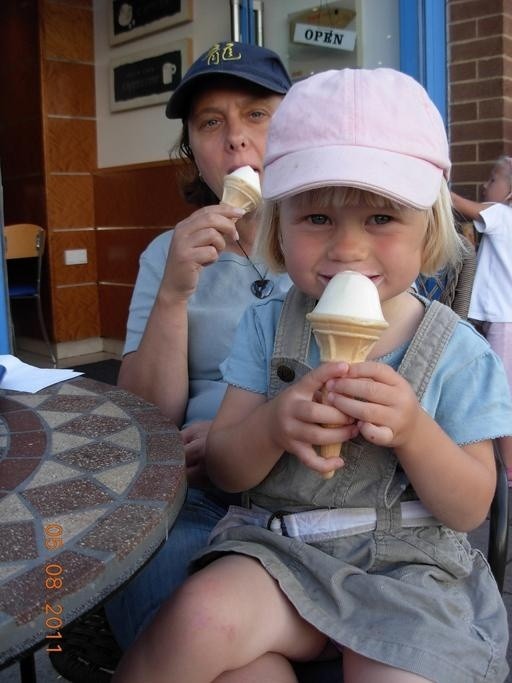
[235,239,274,299]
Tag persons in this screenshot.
[101,42,294,682]
[117,68,510,683]
[449,155,511,482]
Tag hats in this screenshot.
[262,66,451,212]
[164,40,291,120]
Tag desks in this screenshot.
[1,367,189,683]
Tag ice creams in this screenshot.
[219,165,262,226]
[304,270,389,479]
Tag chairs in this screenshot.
[3,223,58,366]
